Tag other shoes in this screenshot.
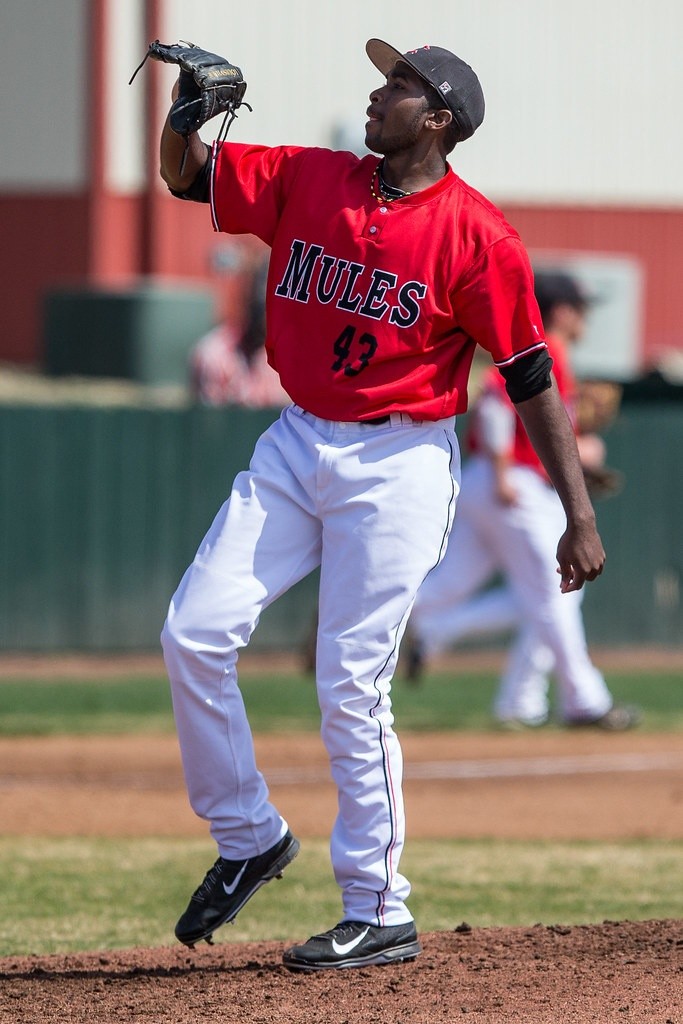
[573,708,642,735]
[501,712,564,737]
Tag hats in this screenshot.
[363,38,486,143]
[534,273,596,308]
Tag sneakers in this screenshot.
[280,921,421,970]
[174,828,302,946]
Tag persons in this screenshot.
[309,273,647,735]
[191,256,294,406]
[151,38,606,969]
